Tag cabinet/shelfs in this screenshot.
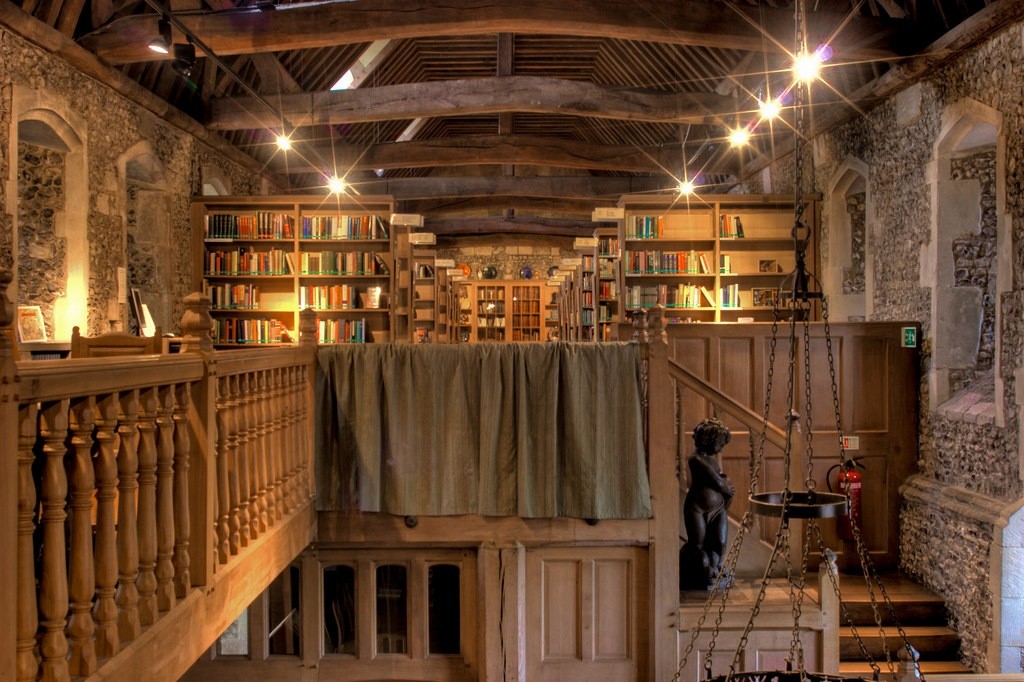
[191,195,823,348]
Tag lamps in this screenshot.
[172,35,195,77]
[148,15,172,53]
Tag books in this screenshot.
[202,212,390,240]
[719,214,744,238]
[477,287,540,341]
[203,246,390,276]
[17,305,48,345]
[206,283,261,310]
[581,237,618,342]
[413,261,434,278]
[625,249,733,275]
[625,281,739,308]
[210,317,366,344]
[299,284,357,310]
[628,212,664,239]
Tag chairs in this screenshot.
[72,326,163,358]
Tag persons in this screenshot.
[679,417,736,585]
[418,327,432,344]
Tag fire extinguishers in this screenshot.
[825,454,867,542]
